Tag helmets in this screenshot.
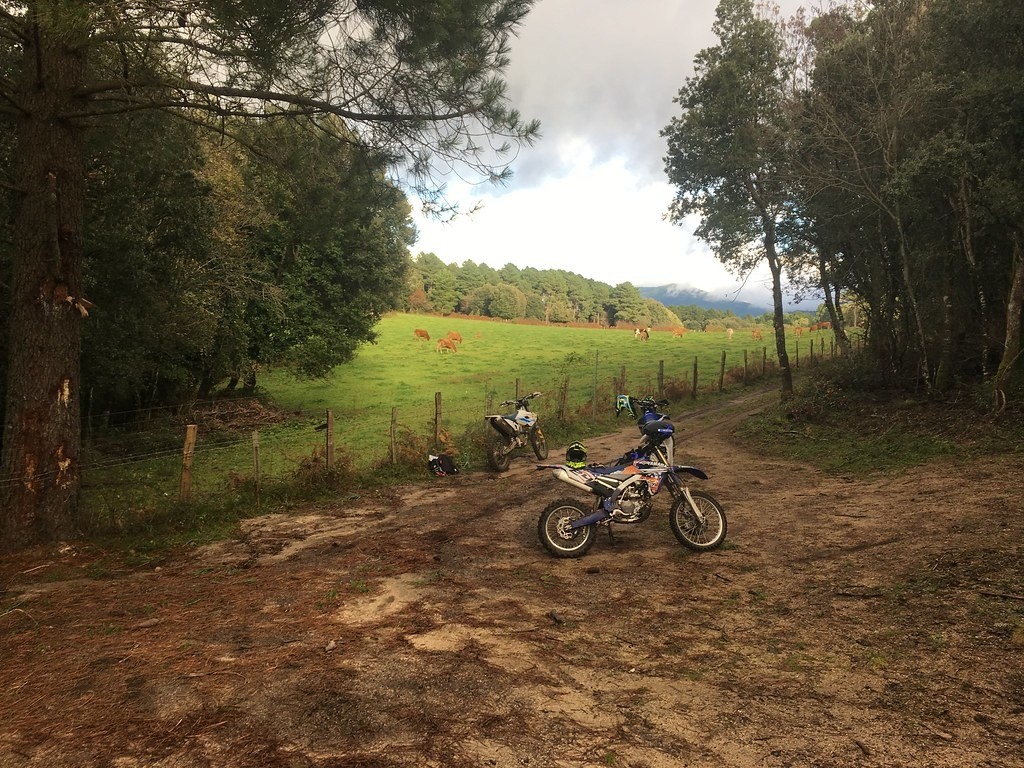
[615,395,638,420]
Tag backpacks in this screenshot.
[428,454,460,477]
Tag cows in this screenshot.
[751,328,763,342]
[436,331,464,354]
[639,332,648,342]
[413,329,430,342]
[795,322,832,337]
[634,324,652,339]
[672,326,690,339]
[726,327,734,340]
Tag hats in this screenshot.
[565,441,587,469]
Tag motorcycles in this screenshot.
[535,421,727,560]
[616,396,676,461]
[484,391,550,471]
